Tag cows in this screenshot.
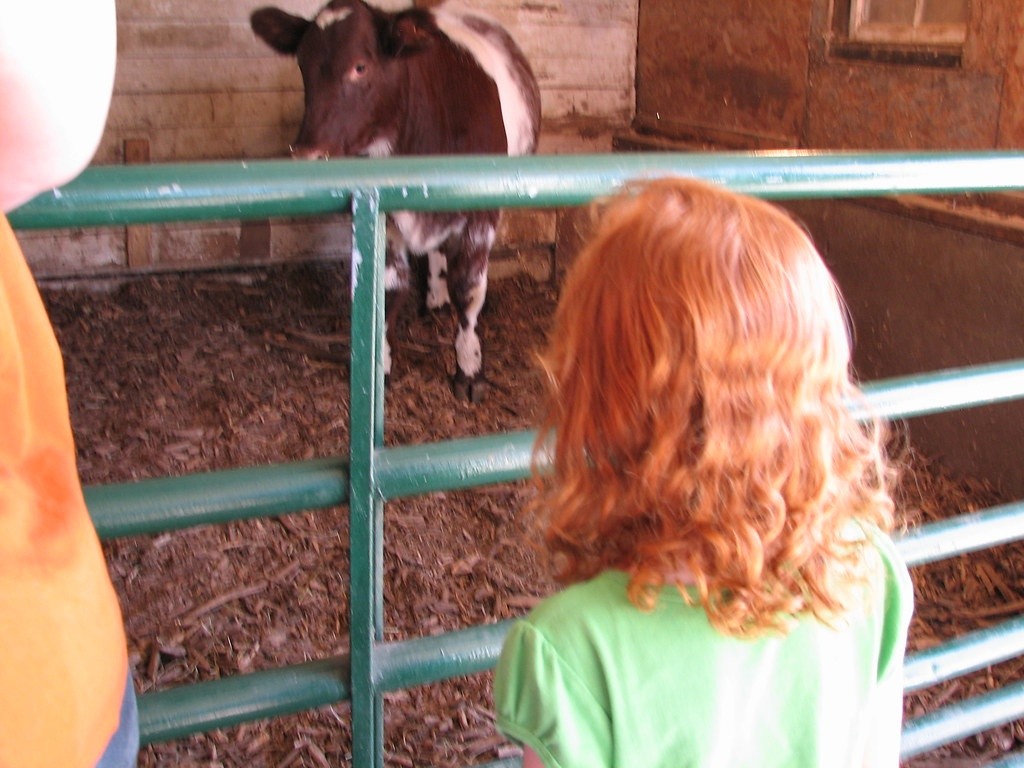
[250,0,543,405]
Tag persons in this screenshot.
[0,1,139,768]
[494,174,915,768]
